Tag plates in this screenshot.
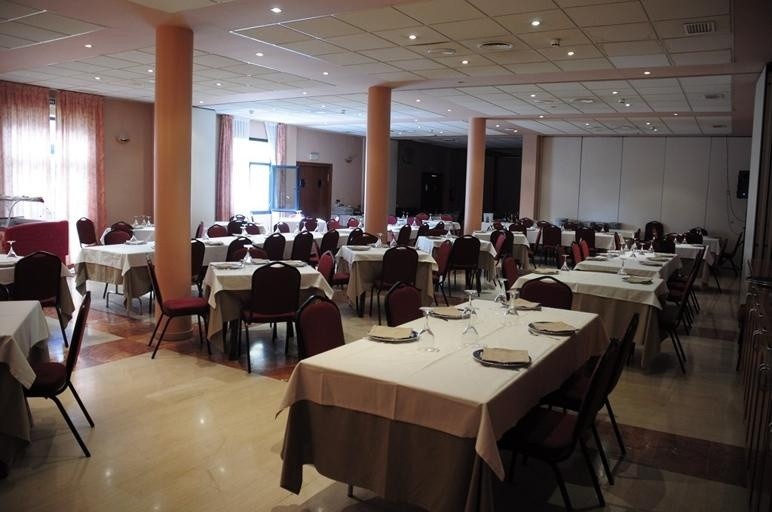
[279,260,307,267]
[531,269,558,275]
[367,326,418,343]
[346,245,370,251]
[501,300,541,311]
[126,240,145,245]
[472,349,532,369]
[242,258,269,264]
[586,257,606,261]
[622,277,653,284]
[529,321,576,336]
[639,254,674,266]
[212,263,242,269]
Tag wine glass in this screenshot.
[445,225,452,239]
[606,238,654,263]
[417,278,519,351]
[374,233,384,248]
[202,228,210,242]
[132,215,152,227]
[240,227,248,238]
[406,213,409,219]
[302,222,307,232]
[617,258,628,278]
[6,241,17,259]
[243,245,253,267]
[130,229,138,241]
[390,233,397,248]
[428,214,433,221]
[560,254,571,274]
[401,212,405,219]
[411,217,418,228]
[276,222,280,233]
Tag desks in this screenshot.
[0,219,703,512]
